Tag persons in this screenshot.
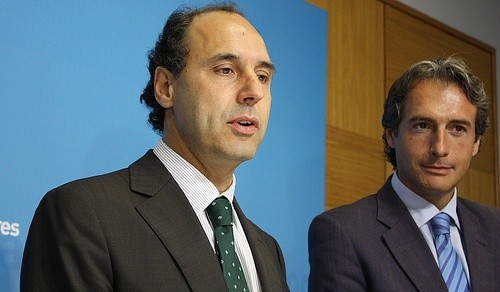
[307,53,500,292]
[20,1,292,292]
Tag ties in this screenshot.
[431,213,471,292]
[208,197,250,292]
